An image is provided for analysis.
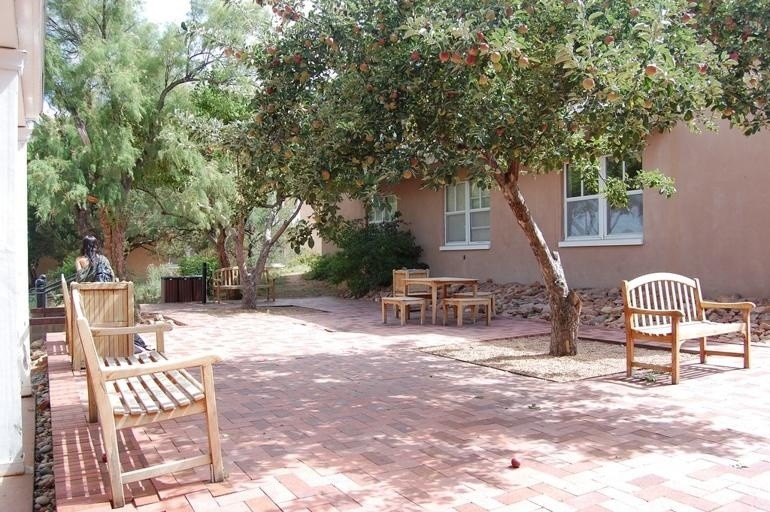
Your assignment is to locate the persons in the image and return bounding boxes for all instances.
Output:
[75,234,152,352]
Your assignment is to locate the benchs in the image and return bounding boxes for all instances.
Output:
[213,265,276,304]
[71,289,225,503]
[622,271,756,384]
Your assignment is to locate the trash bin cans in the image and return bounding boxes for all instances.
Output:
[161,275,204,302]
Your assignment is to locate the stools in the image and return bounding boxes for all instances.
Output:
[381,291,496,328]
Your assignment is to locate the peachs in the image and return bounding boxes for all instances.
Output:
[511,458,520,468]
[223,0,770,188]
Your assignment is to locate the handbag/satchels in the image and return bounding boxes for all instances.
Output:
[92,255,113,282]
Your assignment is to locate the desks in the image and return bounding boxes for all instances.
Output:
[400,277,480,325]
